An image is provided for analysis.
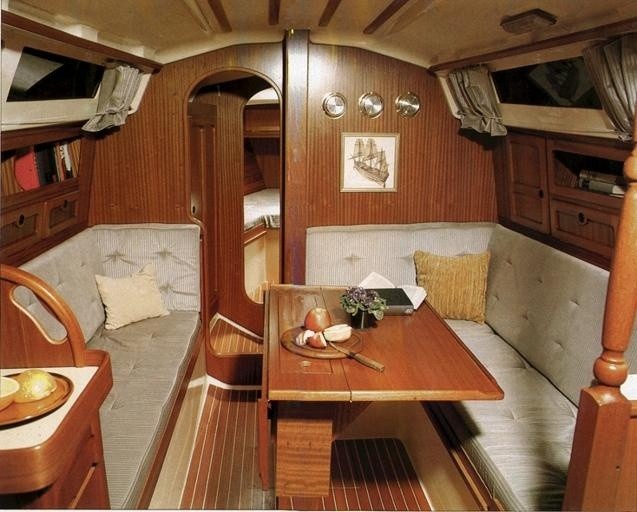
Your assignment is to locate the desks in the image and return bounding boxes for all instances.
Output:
[251,283,504,497]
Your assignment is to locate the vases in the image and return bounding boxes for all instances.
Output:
[347,309,376,329]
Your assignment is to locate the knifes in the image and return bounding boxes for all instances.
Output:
[327,341,385,373]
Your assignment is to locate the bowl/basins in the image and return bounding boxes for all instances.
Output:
[0,376,20,412]
[13,368,58,404]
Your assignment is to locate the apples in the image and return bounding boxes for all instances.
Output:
[304,308,331,333]
[295,323,352,348]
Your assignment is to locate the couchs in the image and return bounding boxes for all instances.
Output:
[0,221,206,509]
[244,187,278,247]
[305,221,636,512]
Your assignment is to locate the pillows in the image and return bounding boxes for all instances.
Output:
[411,249,489,325]
[93,264,171,329]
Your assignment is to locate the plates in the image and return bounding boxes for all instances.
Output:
[0,370,76,428]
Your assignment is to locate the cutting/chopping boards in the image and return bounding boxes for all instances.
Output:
[279,324,365,359]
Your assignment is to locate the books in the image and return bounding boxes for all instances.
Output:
[360,287,413,316]
[579,169,626,196]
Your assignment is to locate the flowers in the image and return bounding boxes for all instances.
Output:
[338,285,389,321]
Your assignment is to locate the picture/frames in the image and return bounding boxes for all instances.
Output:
[340,132,399,193]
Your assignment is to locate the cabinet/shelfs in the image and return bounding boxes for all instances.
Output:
[502,128,550,236]
[546,137,636,261]
[1,118,96,267]
[0,350,115,510]
